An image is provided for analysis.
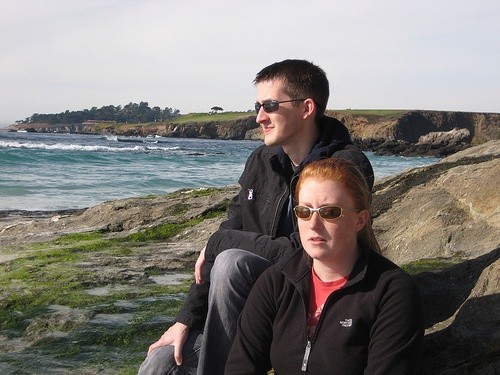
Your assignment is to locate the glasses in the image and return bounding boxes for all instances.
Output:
[293,205,361,220]
[255,98,320,114]
